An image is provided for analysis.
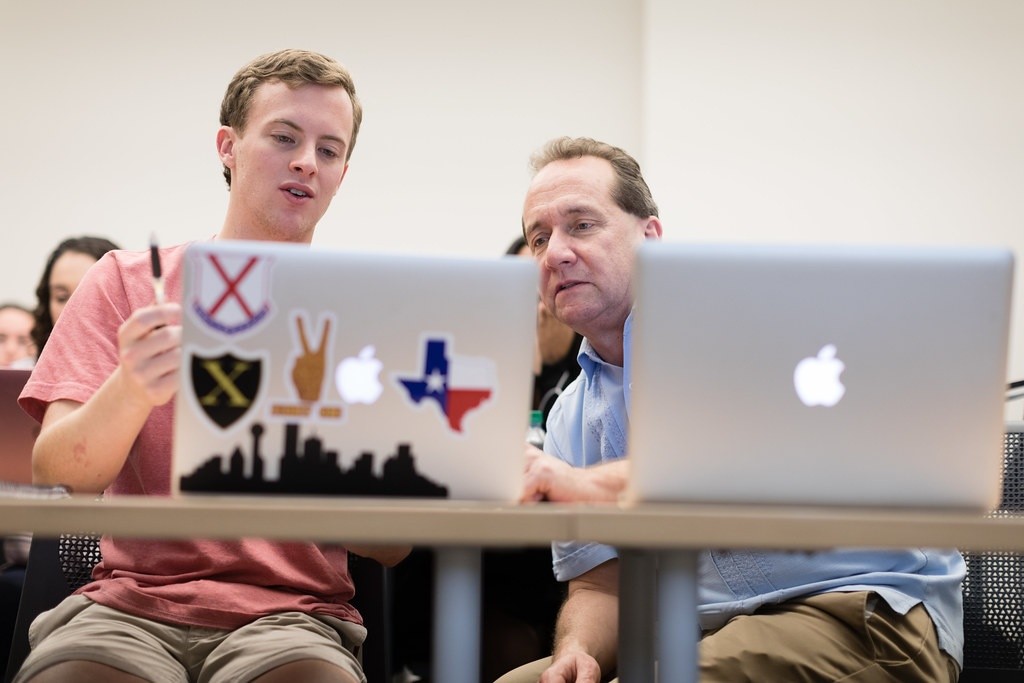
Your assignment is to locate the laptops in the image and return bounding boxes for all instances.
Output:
[0,369,42,487]
[173,245,539,504]
[632,240,1016,511]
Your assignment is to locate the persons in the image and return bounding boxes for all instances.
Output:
[502,237,585,452]
[18,49,417,683]
[30,236,122,369]
[0,305,36,370]
[490,137,969,683]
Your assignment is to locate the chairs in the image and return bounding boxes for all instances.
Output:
[5,532,104,683]
[956,425,1024,683]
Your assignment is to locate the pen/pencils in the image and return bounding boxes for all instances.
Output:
[148,232,167,332]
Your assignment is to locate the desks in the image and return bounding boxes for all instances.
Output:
[0,491,1024,683]
